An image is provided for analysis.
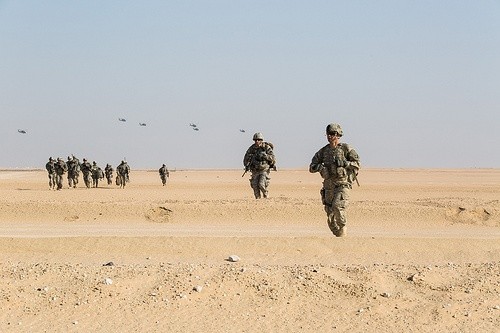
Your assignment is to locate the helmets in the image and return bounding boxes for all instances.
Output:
[327,123,343,136]
[68,156,72,159]
[57,157,61,160]
[49,157,54,160]
[253,133,263,140]
[163,164,166,166]
[122,161,124,164]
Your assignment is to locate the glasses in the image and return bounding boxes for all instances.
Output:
[327,132,339,136]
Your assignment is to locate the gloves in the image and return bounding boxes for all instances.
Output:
[336,159,347,167]
[312,162,324,172]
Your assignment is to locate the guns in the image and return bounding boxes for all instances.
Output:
[242,146,265,177]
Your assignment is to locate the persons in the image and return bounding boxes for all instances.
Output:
[243,132,276,198]
[46,157,56,191]
[55,157,67,190]
[159,163,169,186]
[309,124,359,237]
[66,155,131,189]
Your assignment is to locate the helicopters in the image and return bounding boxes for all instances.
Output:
[189,123,197,127]
[18,128,26,134]
[139,122,146,127]
[240,129,246,132]
[193,127,199,131]
[119,117,126,121]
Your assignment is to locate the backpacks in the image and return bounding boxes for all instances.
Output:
[341,143,360,183]
[263,142,275,167]
